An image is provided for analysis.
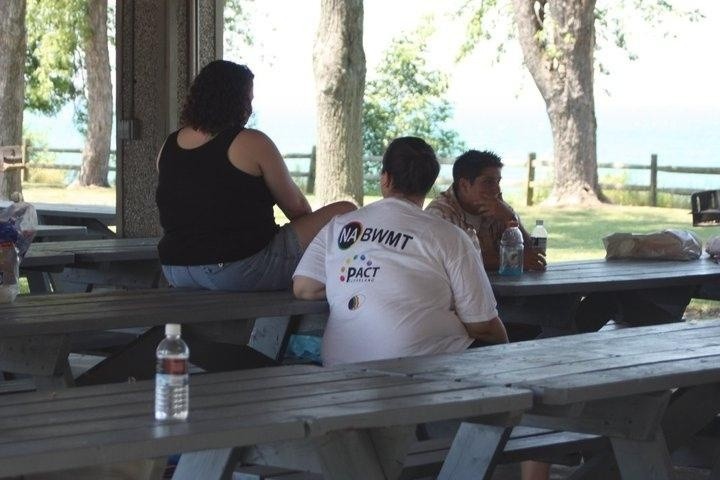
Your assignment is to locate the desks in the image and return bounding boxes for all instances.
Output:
[0,286,336,396]
[331,317,719,480]
[0,364,535,480]
[0,201,116,240]
[486,258,720,338]
[22,238,169,293]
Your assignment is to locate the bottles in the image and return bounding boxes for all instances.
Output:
[466,225,483,263]
[499,218,524,276]
[156,323,190,422]
[529,219,548,272]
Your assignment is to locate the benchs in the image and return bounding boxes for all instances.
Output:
[34,223,87,241]
[72,327,163,363]
[233,425,602,480]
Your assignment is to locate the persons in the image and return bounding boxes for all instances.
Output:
[421,148,548,274]
[156,60,358,293]
[291,136,551,479]
